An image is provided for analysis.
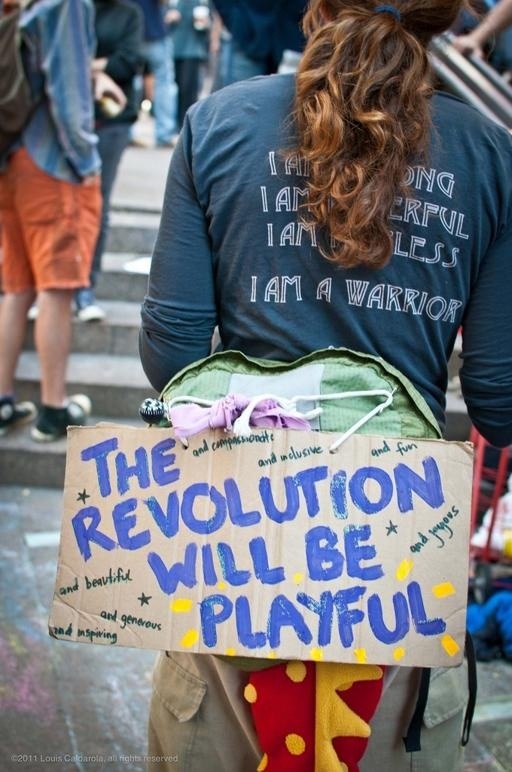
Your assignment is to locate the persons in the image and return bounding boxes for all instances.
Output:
[134,0,512,769]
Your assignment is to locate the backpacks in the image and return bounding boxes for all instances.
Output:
[0,0,41,154]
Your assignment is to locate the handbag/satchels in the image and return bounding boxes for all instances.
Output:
[154,346,443,439]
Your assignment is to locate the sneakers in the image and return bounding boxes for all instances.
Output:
[0,393,91,440]
[78,304,104,324]
